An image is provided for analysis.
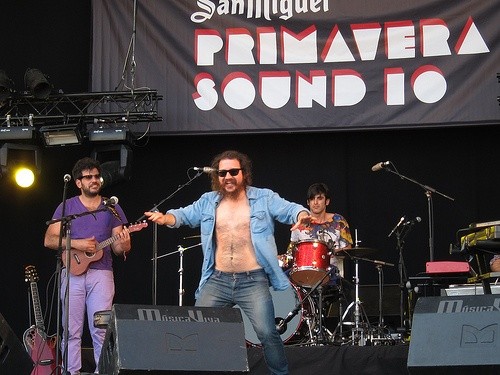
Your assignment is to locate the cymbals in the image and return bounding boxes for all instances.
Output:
[334,247,372,256]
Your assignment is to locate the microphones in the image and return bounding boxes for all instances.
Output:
[275,320,286,333]
[371,161,390,172]
[193,166,216,173]
[403,217,422,225]
[63,173,71,183]
[102,196,119,206]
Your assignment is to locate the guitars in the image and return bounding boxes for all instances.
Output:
[24,265,62,375]
[62,219,148,275]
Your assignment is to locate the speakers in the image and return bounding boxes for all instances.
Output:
[407,293,500,375]
[95,303,251,375]
[0,314,35,375]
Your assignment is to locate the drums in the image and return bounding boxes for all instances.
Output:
[290,239,330,286]
[232,280,317,348]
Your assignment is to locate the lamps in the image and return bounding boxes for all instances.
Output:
[0,69,17,110]
[0,124,136,189]
[23,65,53,97]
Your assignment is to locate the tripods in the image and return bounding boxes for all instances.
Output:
[285,253,372,346]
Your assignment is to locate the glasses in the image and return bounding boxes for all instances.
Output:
[218,169,243,177]
[79,174,100,179]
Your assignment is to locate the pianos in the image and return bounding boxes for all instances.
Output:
[426,261,477,278]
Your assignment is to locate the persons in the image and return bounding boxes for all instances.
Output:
[490,254,500,273]
[283,181,353,325]
[143,149,318,374]
[43,156,132,375]
